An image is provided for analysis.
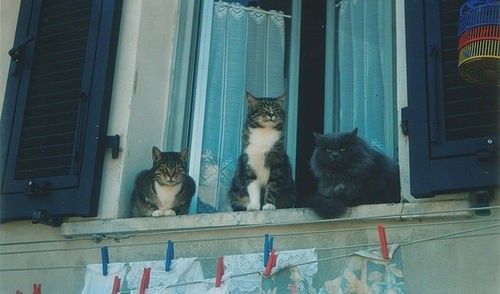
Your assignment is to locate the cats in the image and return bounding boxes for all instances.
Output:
[304,127,401,219]
[130,146,197,218]
[228,90,298,212]
